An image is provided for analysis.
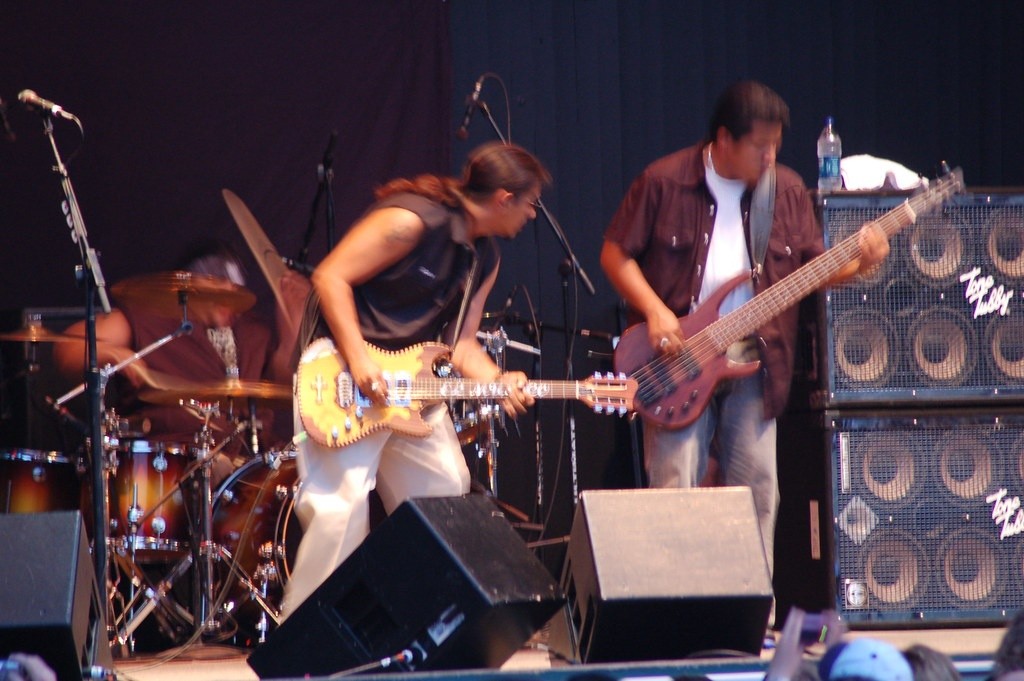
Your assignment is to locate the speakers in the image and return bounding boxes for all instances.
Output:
[246,493,566,681]
[548,486,775,671]
[0,509,117,681]
[806,185,1024,630]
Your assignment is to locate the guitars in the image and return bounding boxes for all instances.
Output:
[296,336,639,450]
[613,161,968,433]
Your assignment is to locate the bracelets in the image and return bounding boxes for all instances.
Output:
[492,368,509,382]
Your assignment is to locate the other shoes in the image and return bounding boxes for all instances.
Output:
[761,626,776,649]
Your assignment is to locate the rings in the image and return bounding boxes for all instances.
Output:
[659,338,668,347]
[371,380,380,392]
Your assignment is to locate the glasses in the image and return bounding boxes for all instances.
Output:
[507,191,541,212]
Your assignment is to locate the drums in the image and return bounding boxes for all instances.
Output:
[90,438,205,563]
[210,448,308,636]
[1,447,89,514]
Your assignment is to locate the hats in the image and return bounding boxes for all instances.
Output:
[818,638,914,681]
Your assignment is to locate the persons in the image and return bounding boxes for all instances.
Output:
[601,77,891,628]
[760,597,1024,681]
[55,253,252,649]
[277,140,552,628]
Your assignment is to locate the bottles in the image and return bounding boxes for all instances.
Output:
[817,119,843,191]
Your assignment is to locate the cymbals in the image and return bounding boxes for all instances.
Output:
[135,380,293,404]
[108,272,255,326]
[0,327,103,348]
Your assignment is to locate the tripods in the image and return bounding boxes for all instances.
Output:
[108,400,284,660]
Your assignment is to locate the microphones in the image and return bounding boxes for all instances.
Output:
[17,89,75,121]
[493,286,519,331]
[46,397,93,435]
[455,74,485,140]
[248,398,259,454]
[280,257,315,276]
